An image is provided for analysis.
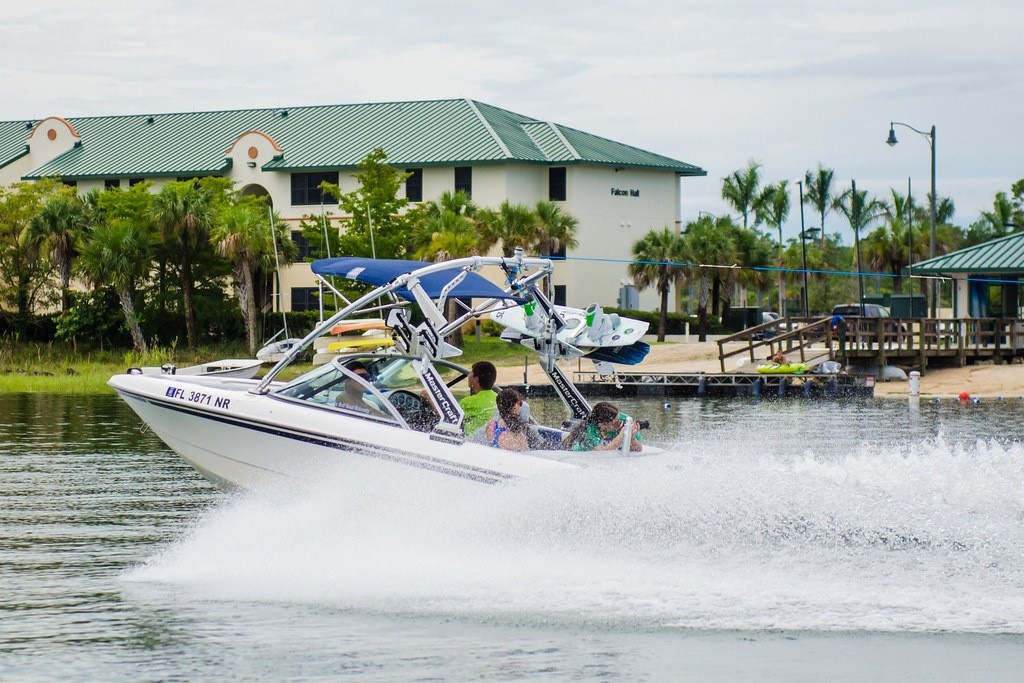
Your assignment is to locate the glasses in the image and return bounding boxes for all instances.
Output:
[358,373,371,381]
[513,401,522,406]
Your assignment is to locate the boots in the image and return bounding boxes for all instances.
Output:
[522,293,543,329]
[585,303,620,340]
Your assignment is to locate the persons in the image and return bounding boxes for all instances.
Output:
[486,388,528,450]
[561,401,643,453]
[422,361,498,436]
[333,361,396,420]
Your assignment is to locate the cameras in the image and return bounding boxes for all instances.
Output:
[636,419,651,429]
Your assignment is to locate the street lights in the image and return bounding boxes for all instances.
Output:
[875,121,936,340]
[795,178,809,319]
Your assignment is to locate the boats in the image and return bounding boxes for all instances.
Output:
[105,201,678,504]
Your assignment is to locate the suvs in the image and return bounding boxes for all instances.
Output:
[833,304,907,343]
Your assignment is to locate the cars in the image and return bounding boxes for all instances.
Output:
[762,312,797,333]
